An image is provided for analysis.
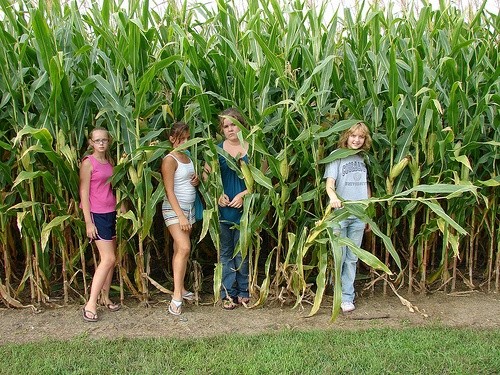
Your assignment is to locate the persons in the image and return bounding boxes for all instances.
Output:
[323,122,373,313]
[161,123,203,317]
[211,108,257,309]
[79,127,128,321]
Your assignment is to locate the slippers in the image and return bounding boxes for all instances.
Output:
[82,307,98,322]
[182,292,202,301]
[101,301,121,312]
[168,298,182,316]
[237,297,250,304]
[223,301,236,310]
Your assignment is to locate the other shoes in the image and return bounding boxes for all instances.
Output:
[340,302,356,313]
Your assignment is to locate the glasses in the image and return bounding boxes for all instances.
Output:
[91,139,110,144]
